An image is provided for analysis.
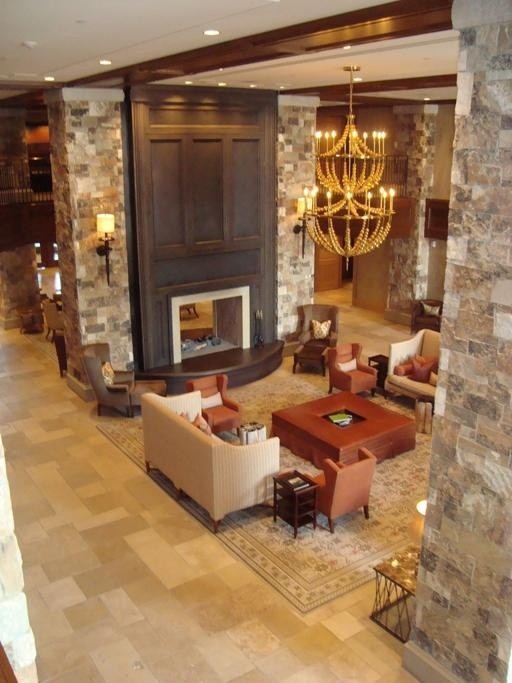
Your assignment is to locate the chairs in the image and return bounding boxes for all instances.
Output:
[412,298,443,333]
[81,346,167,417]
[293,303,339,376]
[306,446,377,533]
[42,298,64,343]
[187,374,242,439]
[327,341,378,395]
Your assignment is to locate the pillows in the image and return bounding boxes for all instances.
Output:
[338,358,358,372]
[423,304,441,317]
[201,392,223,410]
[395,353,437,386]
[102,363,114,386]
[192,414,211,435]
[310,320,331,339]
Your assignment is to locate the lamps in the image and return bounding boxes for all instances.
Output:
[295,196,313,257]
[96,213,115,285]
[296,67,398,273]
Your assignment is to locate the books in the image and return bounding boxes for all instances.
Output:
[339,414,353,426]
[329,413,349,422]
[283,474,310,492]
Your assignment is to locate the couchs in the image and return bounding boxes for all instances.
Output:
[384,328,441,404]
[140,391,281,533]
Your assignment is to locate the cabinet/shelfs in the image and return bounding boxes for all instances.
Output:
[272,469,320,539]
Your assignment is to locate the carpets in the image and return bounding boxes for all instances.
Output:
[94,370,437,614]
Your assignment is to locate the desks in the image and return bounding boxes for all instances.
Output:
[371,546,423,642]
